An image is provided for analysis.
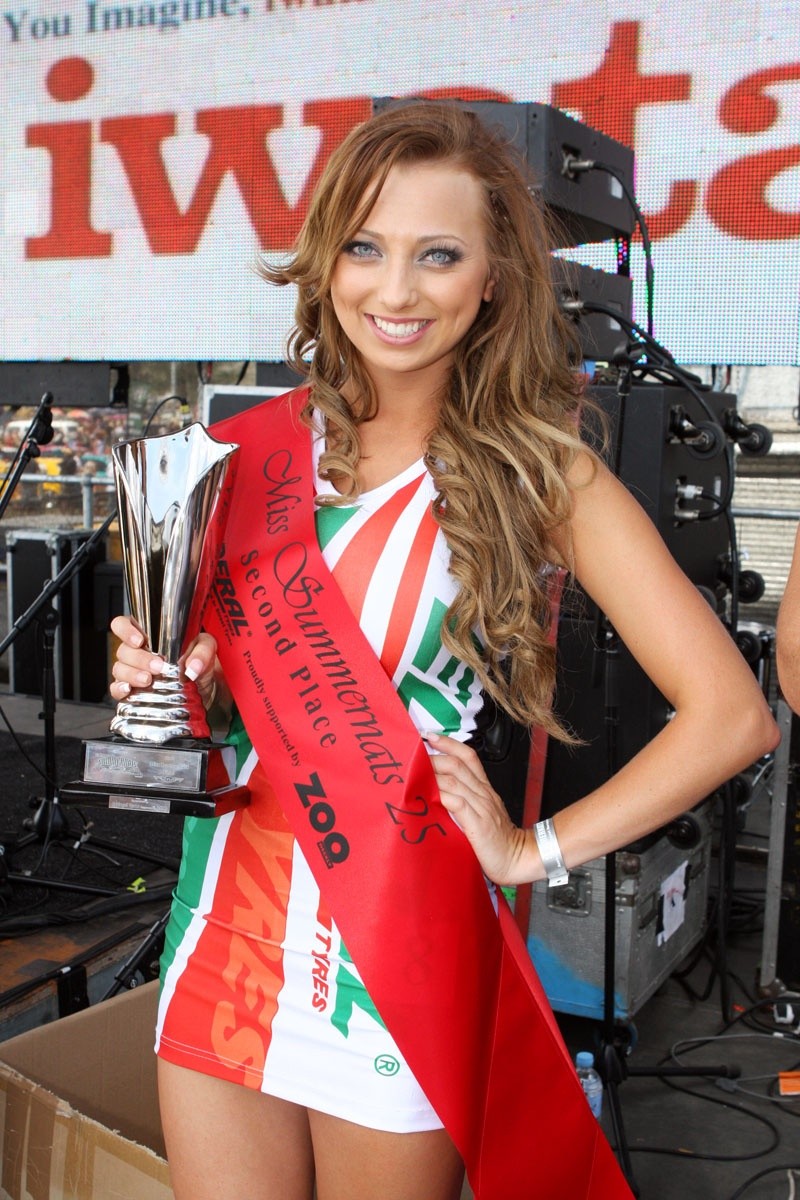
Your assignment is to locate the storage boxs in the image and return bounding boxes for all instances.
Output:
[0,972,180,1200]
[4,523,106,703]
[500,787,713,1026]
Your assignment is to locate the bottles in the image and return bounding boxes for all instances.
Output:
[575,1051,602,1124]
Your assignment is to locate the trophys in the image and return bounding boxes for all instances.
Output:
[59,420,257,818]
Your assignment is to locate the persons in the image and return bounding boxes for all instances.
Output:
[775,525,800,714]
[105,107,781,1200]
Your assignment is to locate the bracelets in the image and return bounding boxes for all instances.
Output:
[533,817,569,890]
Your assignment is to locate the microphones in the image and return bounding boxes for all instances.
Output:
[32,392,54,445]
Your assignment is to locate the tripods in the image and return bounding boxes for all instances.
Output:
[593,851,743,1200]
[0,512,182,880]
